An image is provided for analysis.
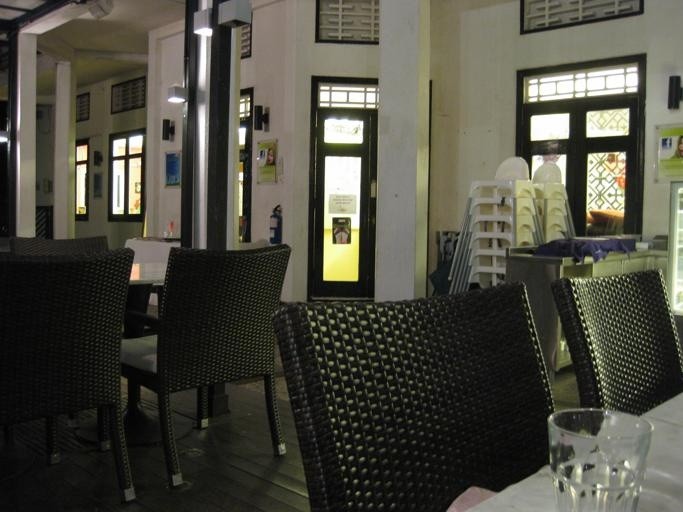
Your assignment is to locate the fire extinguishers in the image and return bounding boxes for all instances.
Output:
[270,204,282,244]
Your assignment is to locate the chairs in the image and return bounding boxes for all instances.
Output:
[0,248,135,502]
[270,279,556,511]
[7,233,109,254]
[547,267,683,417]
[121,243,292,488]
[446,156,577,296]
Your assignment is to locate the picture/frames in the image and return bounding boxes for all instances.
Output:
[75,90,91,124]
[314,2,379,44]
[236,22,252,61]
[517,1,645,36]
[109,73,149,115]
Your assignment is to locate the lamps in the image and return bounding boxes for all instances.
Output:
[91,149,103,168]
[160,118,177,142]
[667,75,682,111]
[223,3,256,36]
[167,83,187,104]
[254,103,270,131]
[192,8,213,42]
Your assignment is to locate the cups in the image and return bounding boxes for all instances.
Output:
[548,408,652,512]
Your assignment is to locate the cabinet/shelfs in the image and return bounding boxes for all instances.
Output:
[507,227,671,372]
